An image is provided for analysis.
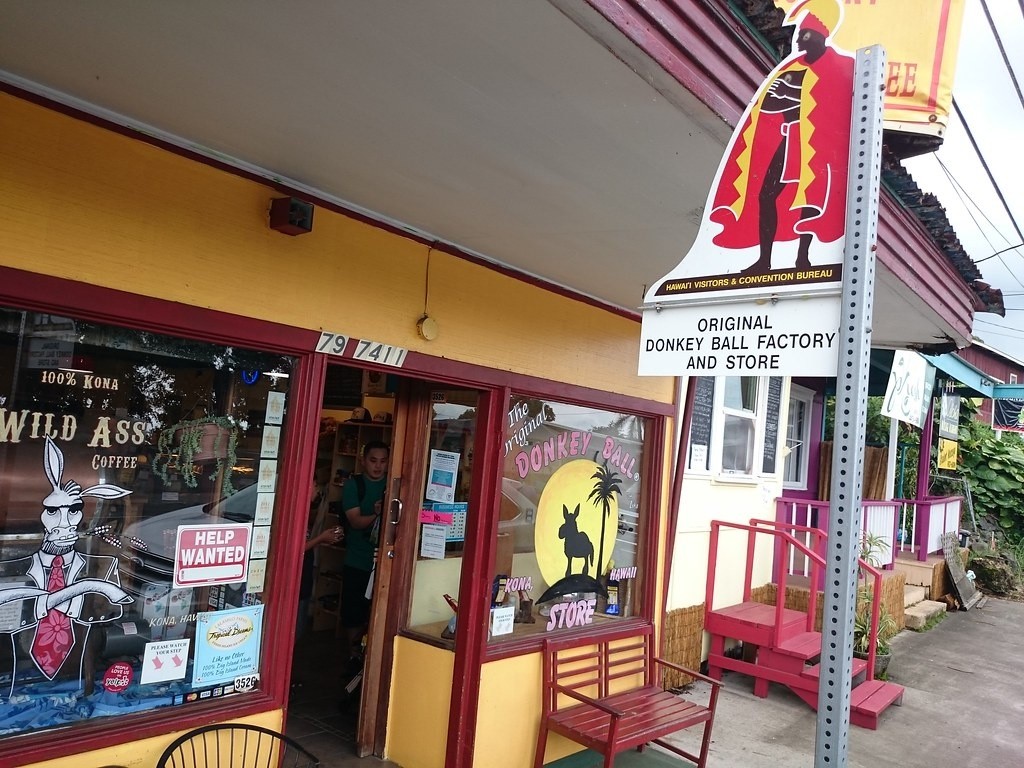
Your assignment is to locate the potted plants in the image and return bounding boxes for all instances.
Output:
[152,415,243,497]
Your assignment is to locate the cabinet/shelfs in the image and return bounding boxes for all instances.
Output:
[311,420,471,639]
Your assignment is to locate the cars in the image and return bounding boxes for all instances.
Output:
[116,482,257,607]
[497,478,542,553]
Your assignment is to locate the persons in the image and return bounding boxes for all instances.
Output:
[132,440,170,522]
[290,471,346,680]
[338,439,390,686]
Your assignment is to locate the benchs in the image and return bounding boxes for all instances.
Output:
[533,621,727,768]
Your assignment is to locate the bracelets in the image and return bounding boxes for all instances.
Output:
[373,512,378,516]
